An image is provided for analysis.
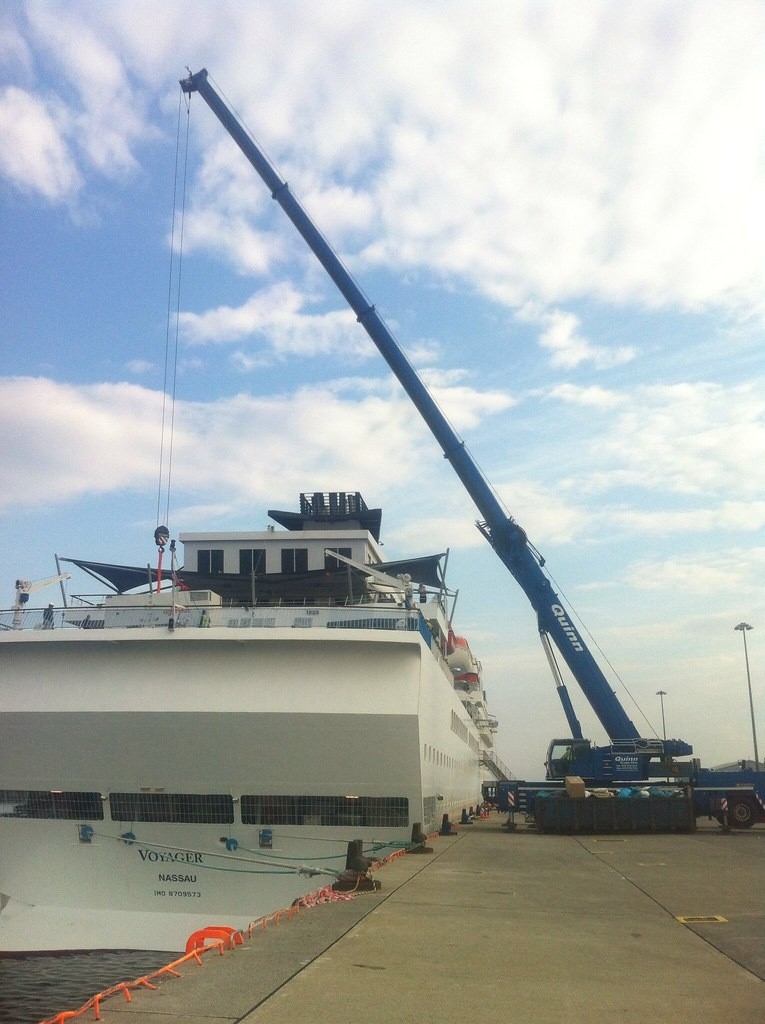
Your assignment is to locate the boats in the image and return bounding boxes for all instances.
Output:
[454,656,482,685]
[1,493,500,959]
[446,636,472,677]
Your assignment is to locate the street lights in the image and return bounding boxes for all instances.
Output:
[734,622,759,773]
[656,690,668,741]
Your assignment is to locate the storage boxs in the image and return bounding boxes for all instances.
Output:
[565,775,585,798]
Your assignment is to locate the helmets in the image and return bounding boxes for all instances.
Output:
[49,602,54,605]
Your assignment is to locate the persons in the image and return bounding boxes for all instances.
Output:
[559,747,572,761]
[417,583,427,603]
[42,604,54,629]
[199,610,212,628]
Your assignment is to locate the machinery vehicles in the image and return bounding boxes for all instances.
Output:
[153,65,765,837]
